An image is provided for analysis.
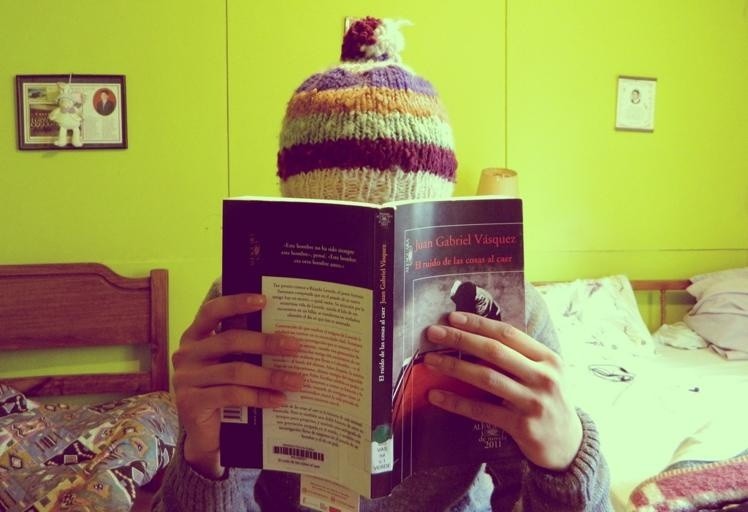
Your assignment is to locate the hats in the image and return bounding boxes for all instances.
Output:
[277,17,457,204]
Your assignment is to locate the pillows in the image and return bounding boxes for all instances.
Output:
[683,266,748,362]
[537,273,657,368]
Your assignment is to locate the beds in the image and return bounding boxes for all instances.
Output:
[0,260,185,512]
[529,278,748,511]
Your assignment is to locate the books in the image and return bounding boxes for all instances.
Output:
[217,193,529,502]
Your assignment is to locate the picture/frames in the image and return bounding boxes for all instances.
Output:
[614,75,658,133]
[14,73,128,151]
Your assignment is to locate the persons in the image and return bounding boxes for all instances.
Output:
[30,112,59,135]
[148,15,618,512]
[97,90,113,114]
